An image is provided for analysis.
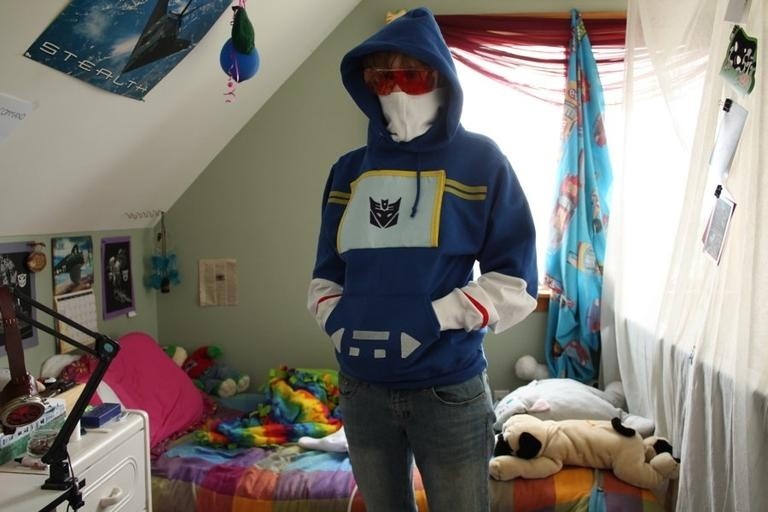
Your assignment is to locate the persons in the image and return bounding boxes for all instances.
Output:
[305,7,538,512]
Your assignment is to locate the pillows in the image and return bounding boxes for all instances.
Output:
[62,331,221,462]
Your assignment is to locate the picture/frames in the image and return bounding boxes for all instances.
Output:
[101,238,136,321]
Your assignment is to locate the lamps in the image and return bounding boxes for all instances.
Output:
[0,285,121,491]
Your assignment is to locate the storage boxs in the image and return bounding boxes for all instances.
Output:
[53,381,87,421]
[0,395,67,468]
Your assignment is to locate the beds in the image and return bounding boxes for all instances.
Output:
[152,376,672,511]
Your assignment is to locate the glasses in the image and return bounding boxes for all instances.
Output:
[363,65,444,96]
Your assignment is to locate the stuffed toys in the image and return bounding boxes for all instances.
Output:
[488,413,681,489]
[493,377,655,440]
[161,344,250,399]
[515,355,549,381]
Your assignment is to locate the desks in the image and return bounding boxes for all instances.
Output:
[0,409,152,512]
